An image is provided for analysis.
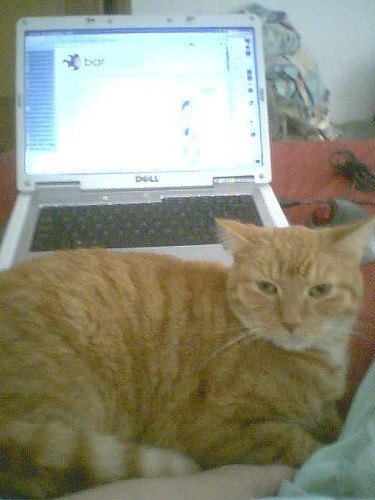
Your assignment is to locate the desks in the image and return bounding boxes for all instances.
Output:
[1,140,374,417]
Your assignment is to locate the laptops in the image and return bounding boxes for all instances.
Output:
[0,15,289,274]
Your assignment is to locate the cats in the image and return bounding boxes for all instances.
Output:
[0,217,374,499]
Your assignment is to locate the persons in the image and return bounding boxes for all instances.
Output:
[59,464,300,500]
